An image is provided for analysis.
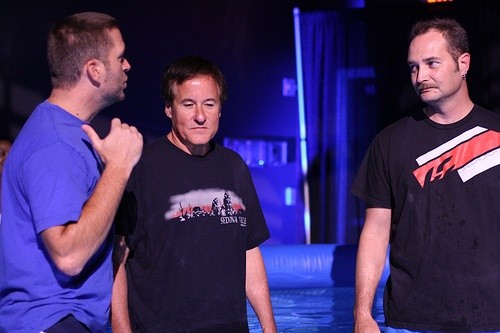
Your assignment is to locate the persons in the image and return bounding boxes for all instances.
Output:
[0,131,17,179]
[349,18,500,333]
[1,11,145,333]
[108,54,278,333]
